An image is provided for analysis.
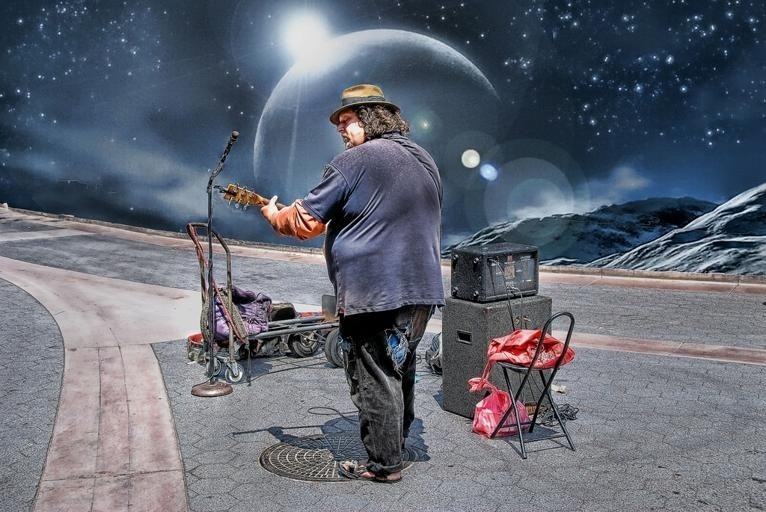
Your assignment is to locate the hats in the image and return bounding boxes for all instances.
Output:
[328,84,401,125]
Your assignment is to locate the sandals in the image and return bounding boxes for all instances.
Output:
[339,460,403,483]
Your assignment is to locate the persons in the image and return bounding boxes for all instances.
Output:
[257,78,447,484]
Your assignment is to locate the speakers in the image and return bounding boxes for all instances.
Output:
[451,241,539,303]
[442,296,552,422]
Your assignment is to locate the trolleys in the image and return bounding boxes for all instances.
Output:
[184,219,350,384]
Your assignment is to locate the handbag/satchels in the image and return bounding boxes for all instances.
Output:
[475,327,575,394]
